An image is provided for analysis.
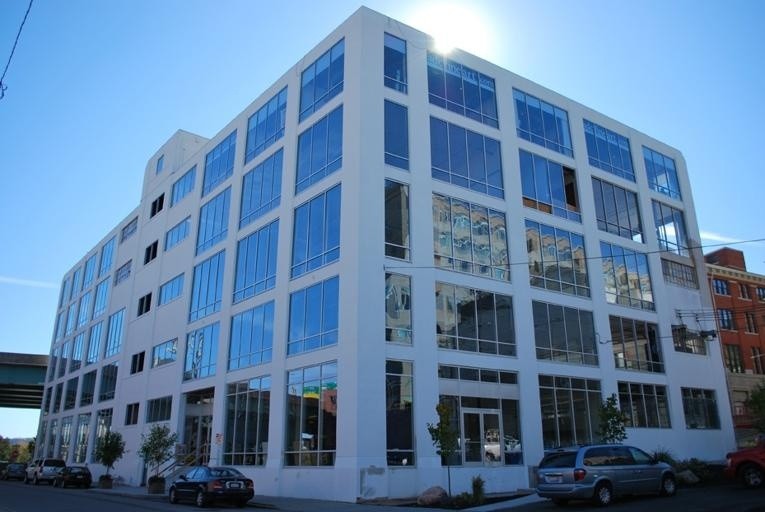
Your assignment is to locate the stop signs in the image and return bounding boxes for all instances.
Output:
[12,451,18,457]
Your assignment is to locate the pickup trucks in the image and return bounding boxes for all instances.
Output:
[458,429,521,461]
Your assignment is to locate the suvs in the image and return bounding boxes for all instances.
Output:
[25,457,66,485]
[535,443,678,505]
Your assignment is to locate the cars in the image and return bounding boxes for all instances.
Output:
[245,447,262,456]
[0,463,24,480]
[0,460,9,469]
[168,466,253,507]
[52,465,91,489]
[722,443,765,488]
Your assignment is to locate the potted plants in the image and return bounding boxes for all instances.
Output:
[136,422,182,494]
[92,428,129,488]
